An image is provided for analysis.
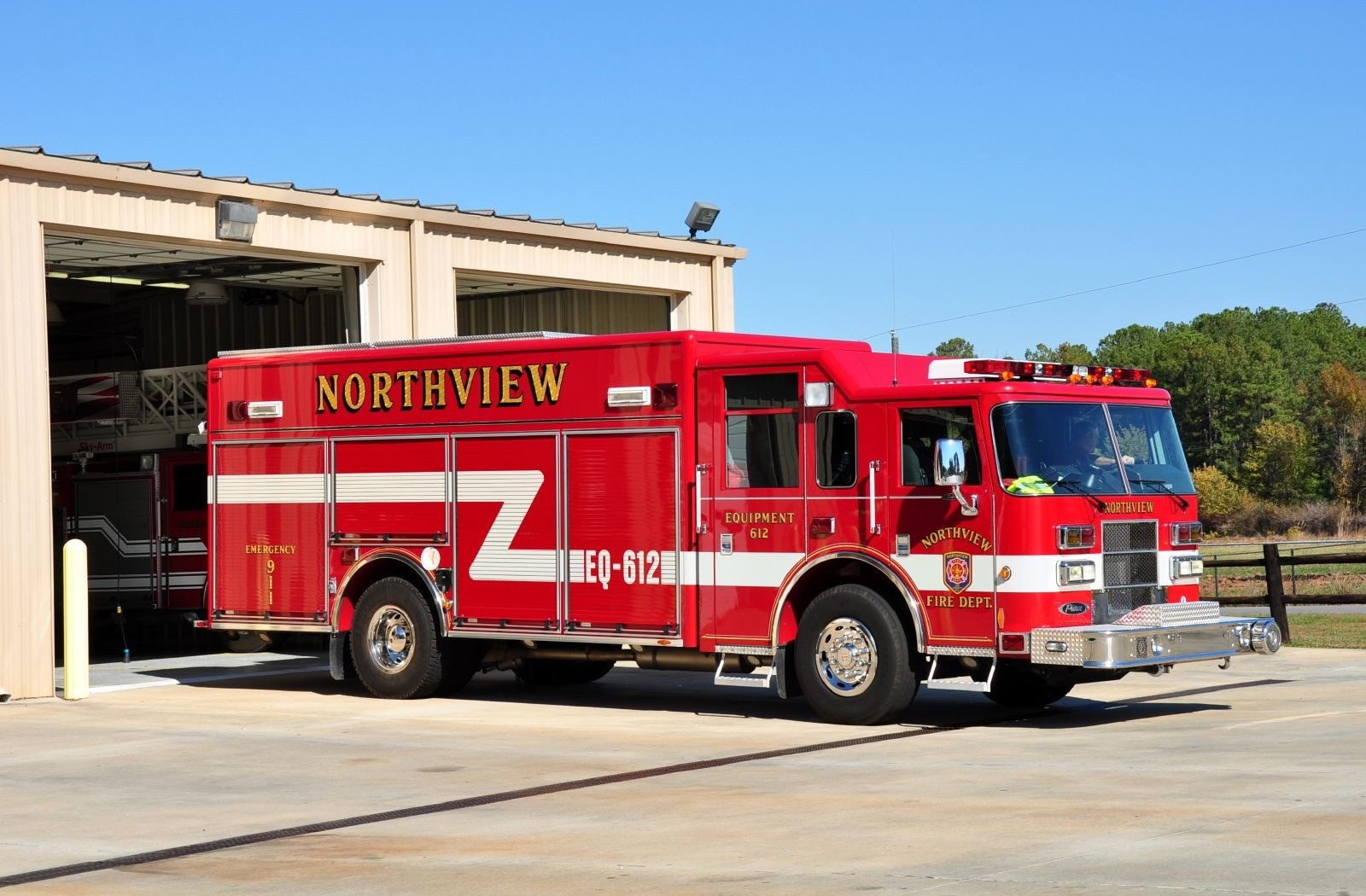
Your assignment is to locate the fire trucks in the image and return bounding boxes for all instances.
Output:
[193,330,1281,727]
[51,364,278,654]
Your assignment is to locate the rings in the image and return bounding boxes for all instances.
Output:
[1126,459,1129,461]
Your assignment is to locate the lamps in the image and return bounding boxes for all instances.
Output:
[216,199,258,243]
[684,201,720,242]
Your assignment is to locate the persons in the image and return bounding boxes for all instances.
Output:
[1059,421,1135,466]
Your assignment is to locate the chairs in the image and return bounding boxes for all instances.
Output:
[969,453,1006,484]
[903,444,925,486]
